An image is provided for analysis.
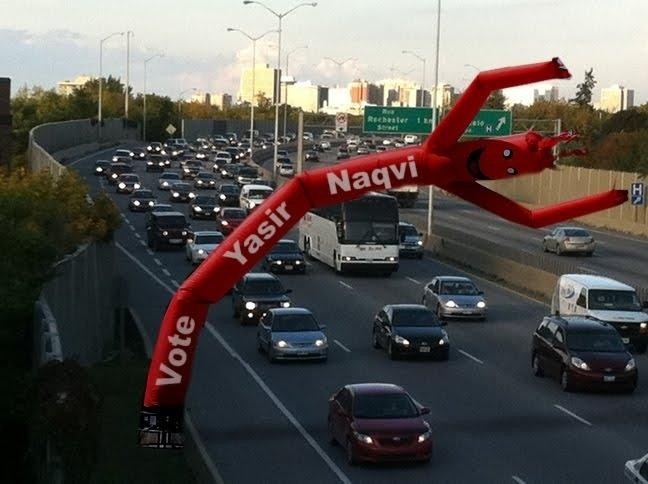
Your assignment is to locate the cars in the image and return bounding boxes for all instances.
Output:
[232,273,293,326]
[327,383,434,465]
[543,226,597,255]
[421,276,488,323]
[371,301,450,362]
[255,305,329,365]
[623,453,648,483]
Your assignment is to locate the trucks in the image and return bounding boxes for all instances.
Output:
[550,274,647,346]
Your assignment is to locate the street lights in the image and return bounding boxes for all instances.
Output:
[228,27,283,160]
[99,31,124,143]
[123,28,138,119]
[388,67,408,106]
[267,44,309,150]
[143,53,165,141]
[243,1,319,186]
[402,49,426,108]
[463,63,481,74]
[178,87,198,134]
[324,55,357,113]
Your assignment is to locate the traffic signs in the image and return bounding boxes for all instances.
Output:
[362,104,439,133]
[442,106,512,137]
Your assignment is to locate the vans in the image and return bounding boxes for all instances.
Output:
[530,313,638,394]
